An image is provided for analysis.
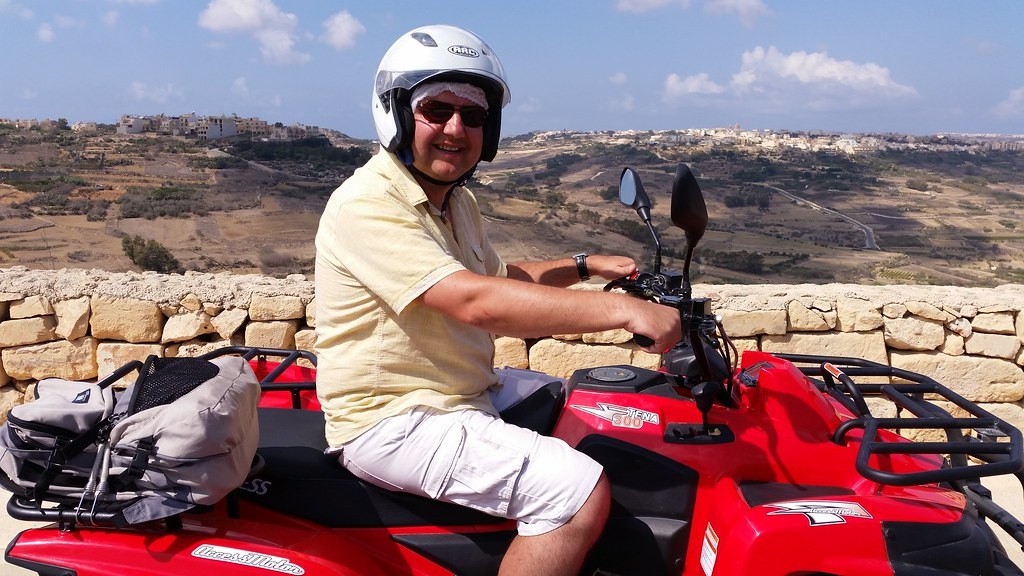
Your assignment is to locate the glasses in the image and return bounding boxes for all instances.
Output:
[418,100,488,129]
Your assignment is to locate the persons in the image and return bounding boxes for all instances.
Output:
[316,24,681,576]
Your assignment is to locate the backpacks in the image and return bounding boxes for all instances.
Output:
[0,354,261,526]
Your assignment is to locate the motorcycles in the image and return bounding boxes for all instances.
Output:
[0,163,1024,576]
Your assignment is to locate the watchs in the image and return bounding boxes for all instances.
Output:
[572,252,591,282]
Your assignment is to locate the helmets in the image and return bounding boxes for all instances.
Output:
[372,24,505,165]
